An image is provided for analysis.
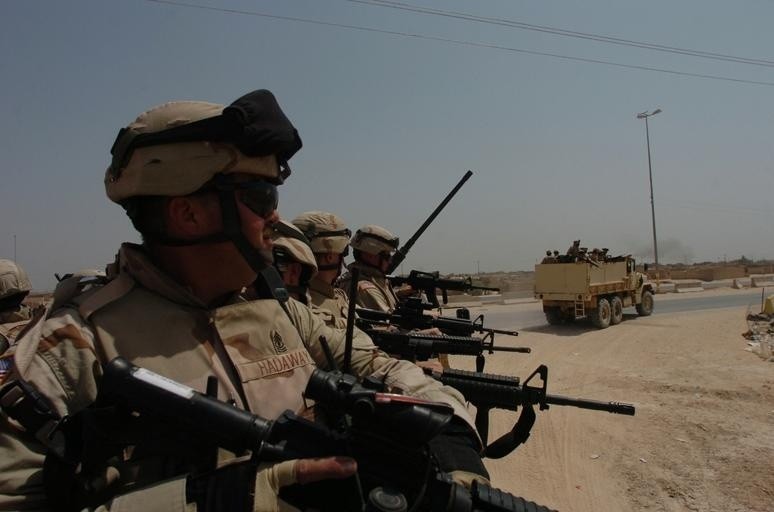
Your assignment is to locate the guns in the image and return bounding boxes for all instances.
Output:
[352,297,521,340]
[348,318,532,359]
[46,356,559,511]
[384,271,502,311]
[415,362,634,415]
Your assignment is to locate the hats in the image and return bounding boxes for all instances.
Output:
[290,210,352,255]
[349,223,400,256]
[104,88,304,205]
[0,256,35,300]
[272,217,319,284]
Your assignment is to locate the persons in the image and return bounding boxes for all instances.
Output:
[541,240,608,264]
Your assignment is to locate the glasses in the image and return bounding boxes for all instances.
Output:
[185,181,278,219]
[273,250,294,266]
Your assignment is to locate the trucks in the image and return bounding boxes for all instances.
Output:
[531,254,655,330]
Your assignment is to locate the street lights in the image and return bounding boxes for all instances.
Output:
[637,110,663,281]
